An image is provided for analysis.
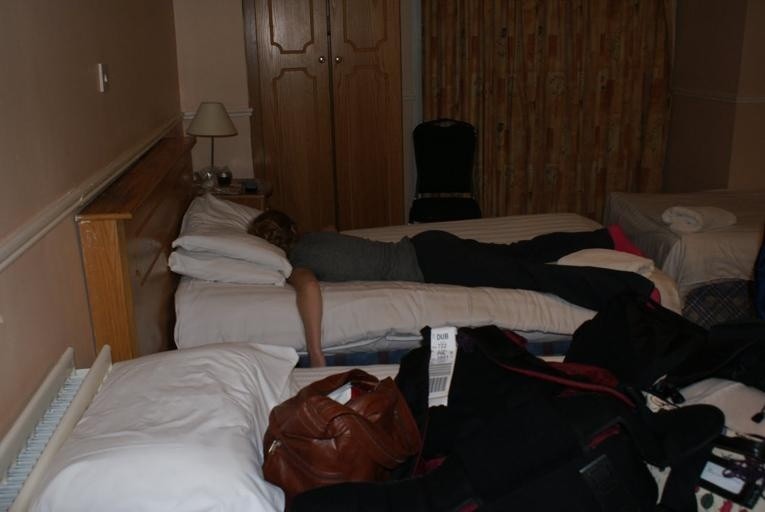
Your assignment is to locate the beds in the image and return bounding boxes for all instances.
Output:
[606,187,765,290]
[75,134,681,356]
[1,342,763,507]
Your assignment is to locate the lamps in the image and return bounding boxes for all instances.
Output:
[189,98,237,184]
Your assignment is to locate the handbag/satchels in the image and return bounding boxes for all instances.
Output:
[262,369,422,496]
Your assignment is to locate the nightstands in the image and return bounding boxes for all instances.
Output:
[198,175,269,211]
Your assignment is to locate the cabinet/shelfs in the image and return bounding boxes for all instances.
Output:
[241,0,406,234]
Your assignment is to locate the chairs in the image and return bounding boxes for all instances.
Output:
[407,117,485,224]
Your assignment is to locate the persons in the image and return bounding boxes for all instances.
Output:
[246,207,662,368]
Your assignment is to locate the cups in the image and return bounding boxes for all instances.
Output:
[214,166,232,186]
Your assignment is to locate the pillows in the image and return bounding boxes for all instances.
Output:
[163,243,286,289]
[174,186,297,270]
[30,340,304,509]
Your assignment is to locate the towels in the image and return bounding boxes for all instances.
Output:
[662,206,737,235]
